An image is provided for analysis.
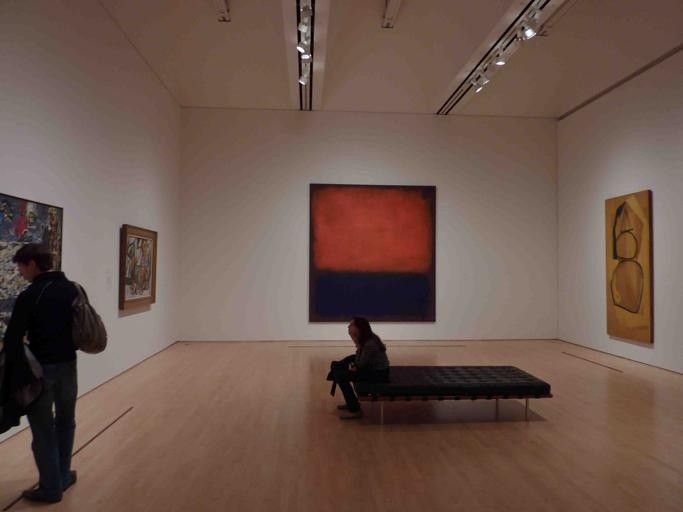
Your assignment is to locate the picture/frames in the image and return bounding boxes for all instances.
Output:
[119,223,158,310]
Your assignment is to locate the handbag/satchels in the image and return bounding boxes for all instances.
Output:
[67,280,107,354]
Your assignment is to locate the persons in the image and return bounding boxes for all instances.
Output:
[335,314,391,421]
[3,243,88,504]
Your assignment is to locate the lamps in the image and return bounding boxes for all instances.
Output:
[296,0,315,111]
[435,0,551,115]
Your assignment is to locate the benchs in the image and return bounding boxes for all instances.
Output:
[357,365,553,425]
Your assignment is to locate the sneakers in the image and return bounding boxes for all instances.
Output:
[62,469,78,492]
[22,487,64,504]
[337,404,351,409]
[340,411,364,419]
